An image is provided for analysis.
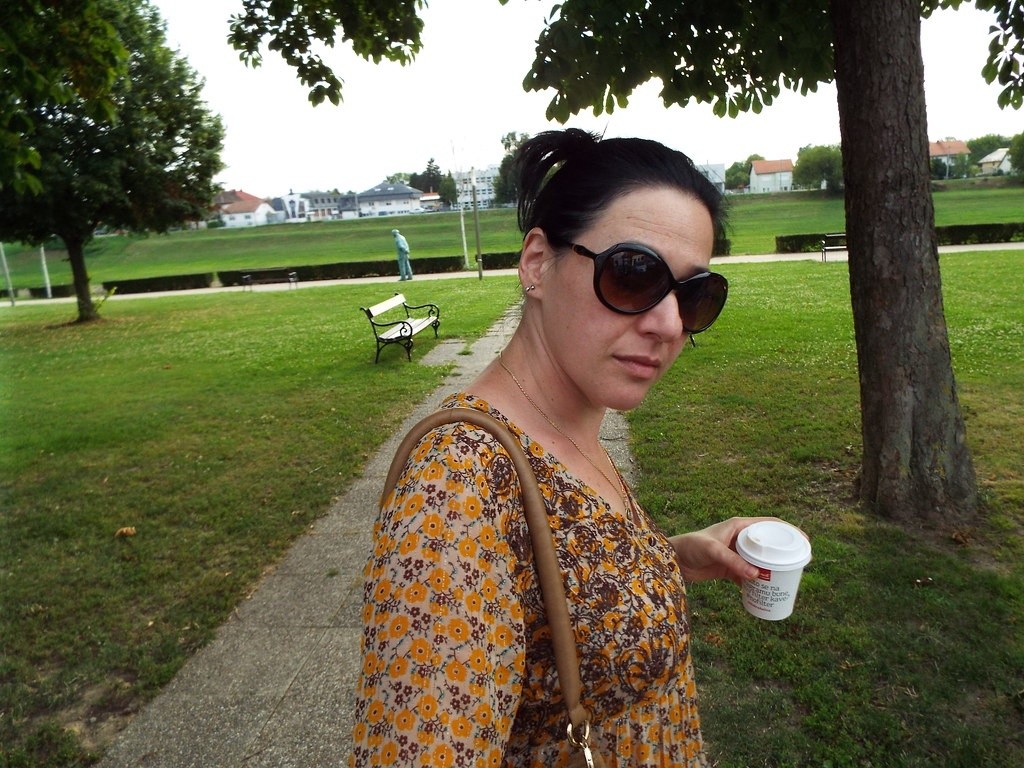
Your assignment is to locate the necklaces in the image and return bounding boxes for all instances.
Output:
[499,352,633,521]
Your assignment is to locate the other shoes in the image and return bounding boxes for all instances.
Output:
[399,279,407,281]
[407,277,413,280]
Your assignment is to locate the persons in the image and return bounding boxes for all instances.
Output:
[351,127,810,768]
[392,229,412,281]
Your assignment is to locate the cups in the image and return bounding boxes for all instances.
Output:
[735,521,812,621]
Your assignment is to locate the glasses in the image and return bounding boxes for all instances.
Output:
[548,239,729,334]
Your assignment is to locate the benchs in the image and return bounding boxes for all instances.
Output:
[360,292,440,364]
[822,234,847,263]
[239,267,300,293]
